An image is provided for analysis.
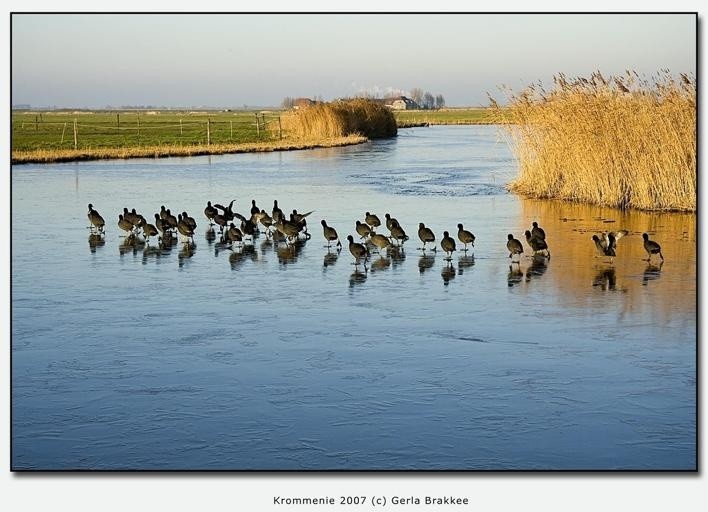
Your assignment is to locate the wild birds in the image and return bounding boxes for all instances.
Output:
[370,253,391,278]
[507,222,546,259]
[643,233,664,260]
[118,206,197,241]
[591,230,628,256]
[642,260,664,286]
[458,224,476,250]
[418,249,435,275]
[458,249,475,275]
[418,223,435,249]
[324,247,342,273]
[88,203,105,233]
[321,220,341,247]
[441,258,456,287]
[508,260,523,287]
[204,200,315,244]
[441,231,456,258]
[347,212,408,264]
[348,264,368,296]
